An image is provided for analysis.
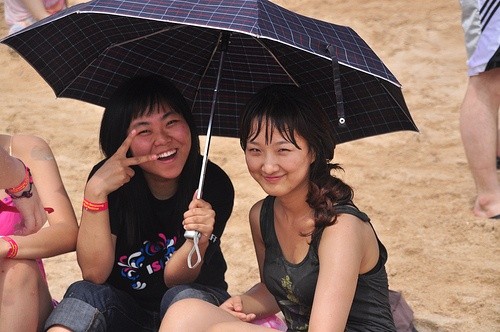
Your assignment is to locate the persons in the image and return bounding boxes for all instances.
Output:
[0,147,48,236]
[460,0,500,220]
[4,0,68,52]
[0,135,79,331]
[43,78,235,331]
[159,84,396,332]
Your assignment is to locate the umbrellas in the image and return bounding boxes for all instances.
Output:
[0,0,421,270]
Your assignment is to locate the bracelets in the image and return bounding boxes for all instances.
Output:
[8,158,28,193]
[82,199,109,212]
[2,236,18,258]
[5,166,33,199]
